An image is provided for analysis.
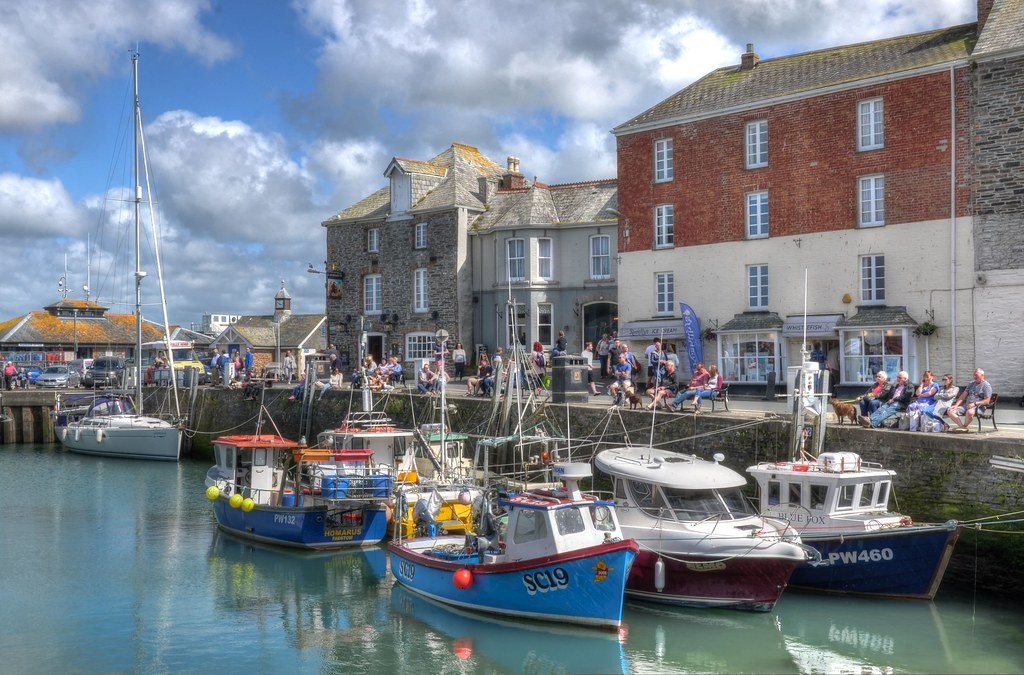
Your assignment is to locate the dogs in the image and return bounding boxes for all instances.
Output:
[624,391,642,410]
[827,398,858,425]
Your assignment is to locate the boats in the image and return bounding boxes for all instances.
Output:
[455,241,594,514]
[387,323,635,632]
[205,381,396,552]
[295,317,491,541]
[585,325,821,612]
[672,266,964,601]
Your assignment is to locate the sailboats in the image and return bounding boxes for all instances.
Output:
[50,40,183,461]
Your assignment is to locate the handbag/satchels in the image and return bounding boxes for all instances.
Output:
[881,411,903,428]
[630,355,641,372]
[899,409,942,432]
[541,372,550,388]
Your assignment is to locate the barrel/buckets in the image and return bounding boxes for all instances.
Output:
[320,475,350,498]
[367,475,393,497]
[281,494,304,507]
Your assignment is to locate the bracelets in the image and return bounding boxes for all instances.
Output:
[974,402,977,406]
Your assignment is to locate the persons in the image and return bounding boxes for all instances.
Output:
[284,350,295,384]
[2,360,19,391]
[209,346,254,387]
[145,356,170,387]
[349,331,568,400]
[288,343,344,403]
[581,321,722,414]
[18,367,29,390]
[811,342,993,434]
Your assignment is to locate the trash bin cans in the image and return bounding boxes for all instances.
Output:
[305,353,331,384]
[552,355,589,402]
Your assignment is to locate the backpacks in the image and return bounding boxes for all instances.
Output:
[533,351,546,371]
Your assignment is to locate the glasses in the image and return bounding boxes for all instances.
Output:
[897,376,902,379]
[618,357,621,358]
[942,379,948,381]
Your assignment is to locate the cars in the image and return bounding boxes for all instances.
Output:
[66,358,94,386]
[35,365,81,388]
[85,355,124,389]
[0,365,43,390]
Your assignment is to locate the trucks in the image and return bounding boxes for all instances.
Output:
[134,339,205,385]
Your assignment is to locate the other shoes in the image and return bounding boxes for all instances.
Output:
[952,427,969,433]
[645,406,651,411]
[665,402,675,412]
[537,386,541,394]
[318,397,321,400]
[691,402,698,405]
[858,415,871,428]
[594,392,601,396]
[462,393,472,396]
[695,411,703,414]
[289,395,295,400]
[944,423,950,432]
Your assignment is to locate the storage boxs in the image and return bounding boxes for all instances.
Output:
[433,549,500,565]
[368,474,394,497]
[281,494,303,507]
[322,475,349,498]
[343,513,361,527]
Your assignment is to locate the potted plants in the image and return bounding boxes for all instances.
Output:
[701,327,716,342]
[913,321,938,340]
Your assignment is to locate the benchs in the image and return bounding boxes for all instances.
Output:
[680,382,730,412]
[472,375,496,395]
[902,393,998,432]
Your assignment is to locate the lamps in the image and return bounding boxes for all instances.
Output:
[308,268,327,275]
[606,207,629,238]
